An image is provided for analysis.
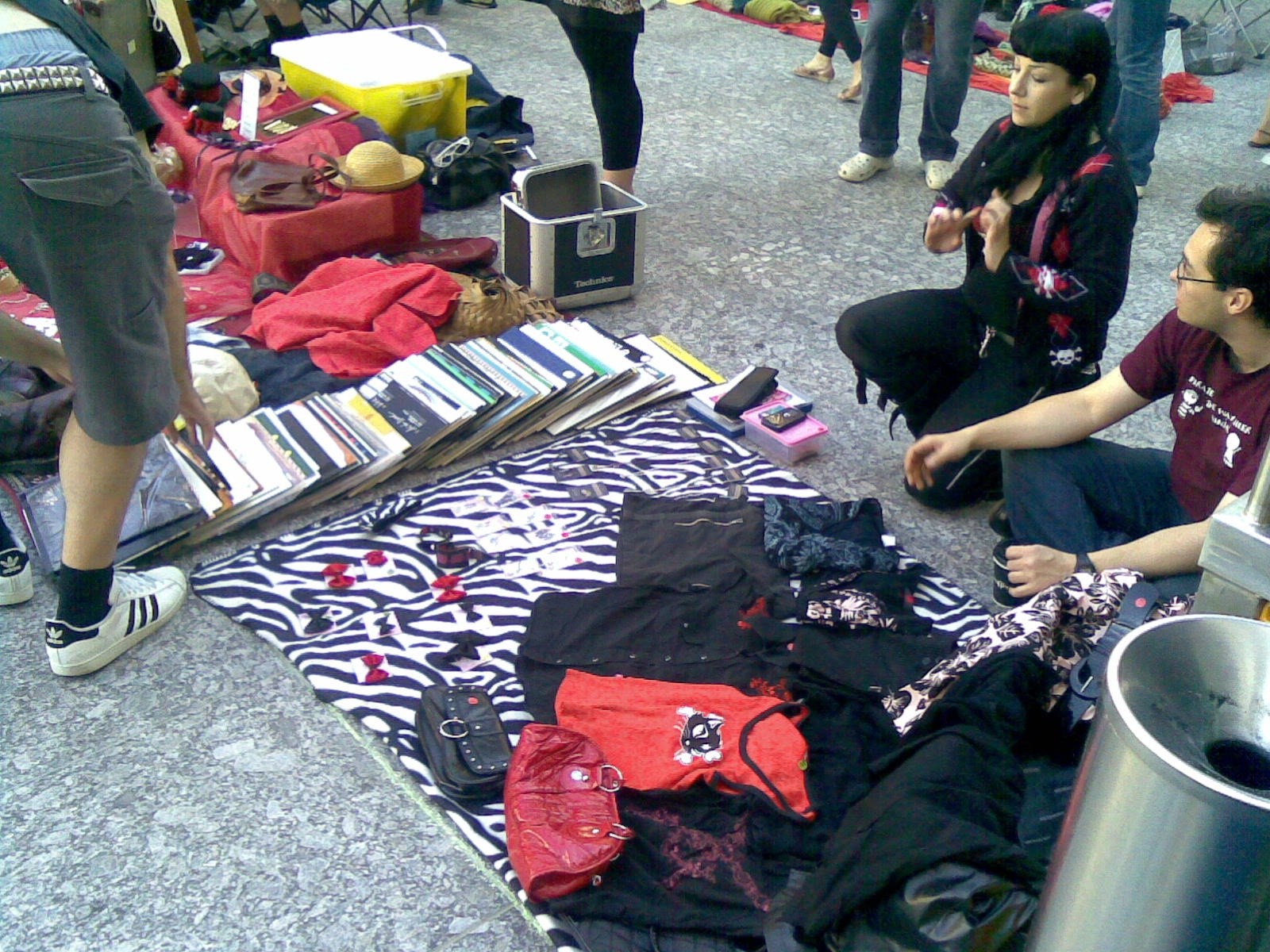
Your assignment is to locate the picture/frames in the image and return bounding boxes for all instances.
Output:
[257,95,359,146]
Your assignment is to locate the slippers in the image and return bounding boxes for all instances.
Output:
[1248,141,1270,149]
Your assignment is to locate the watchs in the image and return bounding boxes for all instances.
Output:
[1074,551,1097,573]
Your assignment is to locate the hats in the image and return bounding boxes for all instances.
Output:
[324,141,425,193]
[228,68,282,109]
[165,62,232,108]
[182,103,232,140]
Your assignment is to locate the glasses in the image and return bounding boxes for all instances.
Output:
[434,137,470,167]
[1176,258,1228,286]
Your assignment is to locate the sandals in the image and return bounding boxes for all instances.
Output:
[838,150,894,183]
[922,158,956,189]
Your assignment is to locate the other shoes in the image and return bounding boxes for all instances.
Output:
[838,78,863,100]
[988,497,1012,538]
[1135,184,1145,197]
[793,64,835,82]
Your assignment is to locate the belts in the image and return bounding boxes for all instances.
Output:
[0,65,111,95]
[1048,583,1161,749]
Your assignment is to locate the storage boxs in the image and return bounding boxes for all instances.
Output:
[500,157,647,310]
[272,28,473,154]
[740,400,828,465]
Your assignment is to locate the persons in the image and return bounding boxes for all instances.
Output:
[904,184,1269,601]
[793,0,864,101]
[834,11,1138,511]
[0,1,215,676]
[1101,0,1172,197]
[250,0,311,67]
[549,0,644,195]
[1248,92,1270,150]
[838,0,986,190]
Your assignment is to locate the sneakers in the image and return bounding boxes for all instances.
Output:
[0,527,34,605]
[235,29,284,67]
[45,566,186,677]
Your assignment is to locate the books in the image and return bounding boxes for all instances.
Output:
[18,315,813,578]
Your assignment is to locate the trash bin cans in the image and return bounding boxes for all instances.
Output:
[1028,613,1270,951]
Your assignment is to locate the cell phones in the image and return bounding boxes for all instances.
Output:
[762,407,806,432]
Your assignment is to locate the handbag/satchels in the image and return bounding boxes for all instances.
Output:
[419,135,508,210]
[228,150,350,212]
[415,684,513,800]
[504,724,635,901]
